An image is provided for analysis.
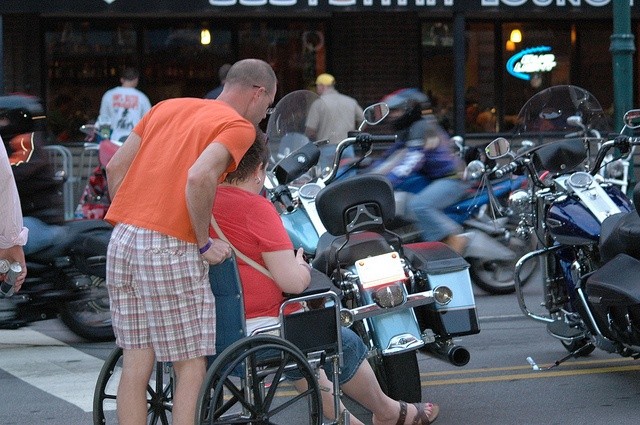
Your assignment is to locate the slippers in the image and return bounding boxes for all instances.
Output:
[397,401,438,424]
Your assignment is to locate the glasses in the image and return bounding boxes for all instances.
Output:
[252,85,277,114]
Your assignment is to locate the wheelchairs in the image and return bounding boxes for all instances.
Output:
[93,240,353,425]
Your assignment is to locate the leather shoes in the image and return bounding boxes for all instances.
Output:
[445,237,470,256]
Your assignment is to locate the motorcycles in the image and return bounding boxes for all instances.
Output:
[320,157,541,296]
[0,217,116,341]
[563,115,640,198]
[70,122,126,221]
[253,87,482,404]
[486,82,640,371]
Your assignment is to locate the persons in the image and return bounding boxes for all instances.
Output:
[303,75,365,178]
[375,88,474,259]
[85,64,152,168]
[0,134,29,294]
[203,62,234,99]
[209,132,439,425]
[104,59,279,425]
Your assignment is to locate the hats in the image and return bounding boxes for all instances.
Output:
[316,73,335,85]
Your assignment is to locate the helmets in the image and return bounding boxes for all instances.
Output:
[384,89,432,131]
